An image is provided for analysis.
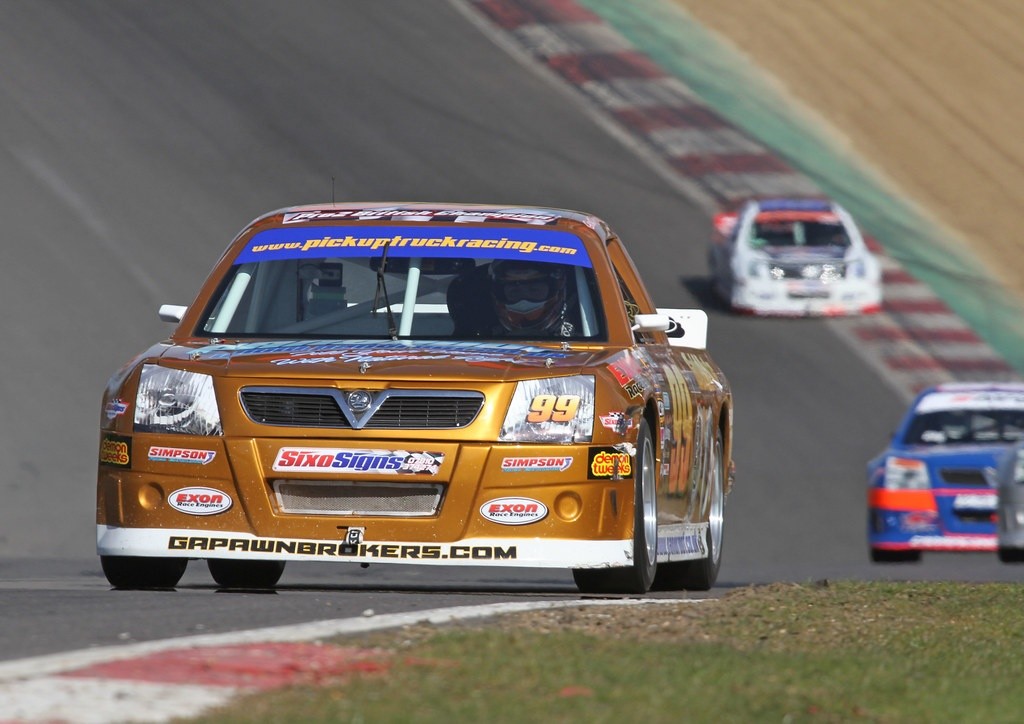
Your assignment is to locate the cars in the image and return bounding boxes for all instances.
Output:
[95,203,732,593]
[866,381,1024,564]
[707,198,884,320]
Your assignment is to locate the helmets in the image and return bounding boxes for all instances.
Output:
[487,259,576,334]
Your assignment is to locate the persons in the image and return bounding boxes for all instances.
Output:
[478,255,583,338]
